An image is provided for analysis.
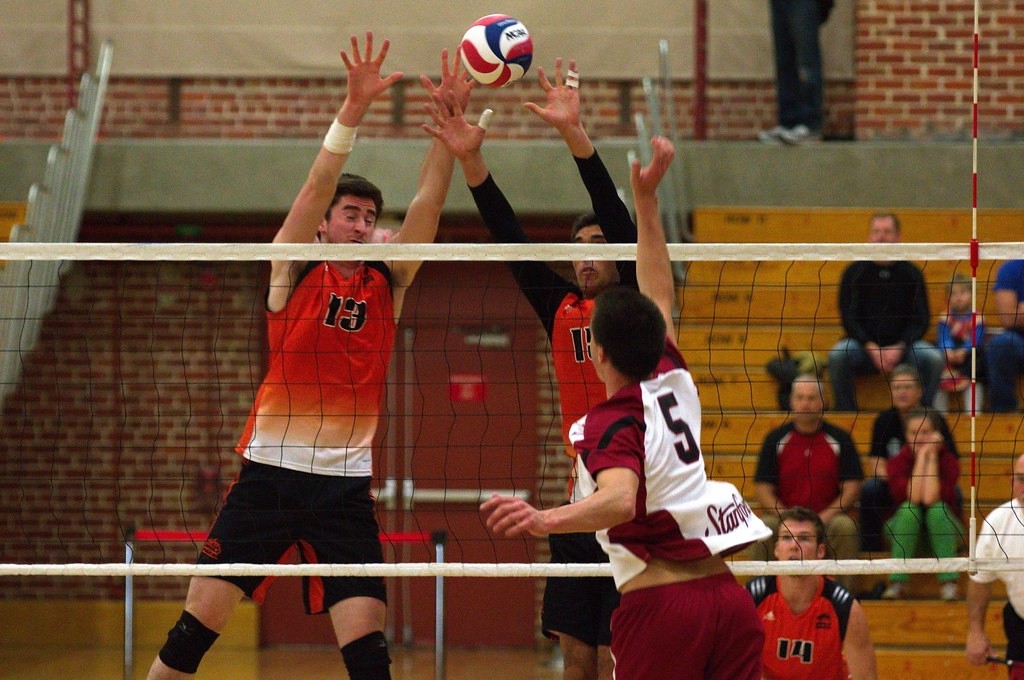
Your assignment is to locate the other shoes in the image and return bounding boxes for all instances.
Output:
[883,583,900,598]
[758,125,789,144]
[941,582,957,600]
[779,125,818,144]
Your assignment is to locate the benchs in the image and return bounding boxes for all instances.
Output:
[675,206,1024,680]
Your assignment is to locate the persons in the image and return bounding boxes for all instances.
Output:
[480,134,774,680]
[757,0,835,144]
[146,30,474,680]
[421,58,637,680]
[967,454,1024,680]
[743,507,877,680]
[751,207,1024,599]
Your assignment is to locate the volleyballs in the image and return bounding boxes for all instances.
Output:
[459,13,533,89]
